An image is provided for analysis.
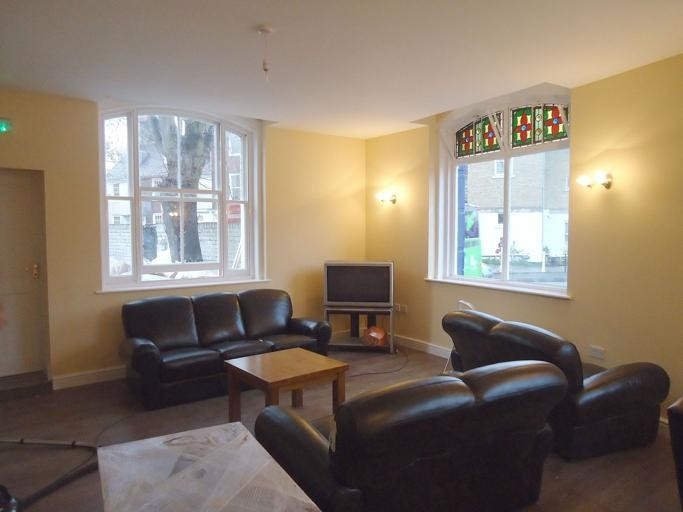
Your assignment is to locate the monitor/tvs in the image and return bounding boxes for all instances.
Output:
[322,260,395,307]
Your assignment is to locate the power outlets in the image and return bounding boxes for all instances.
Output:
[394,304,400,312]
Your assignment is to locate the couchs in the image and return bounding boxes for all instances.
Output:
[254,358,571,512]
[119,288,335,414]
[441,311,671,464]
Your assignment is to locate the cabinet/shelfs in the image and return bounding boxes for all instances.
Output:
[323,307,394,355]
